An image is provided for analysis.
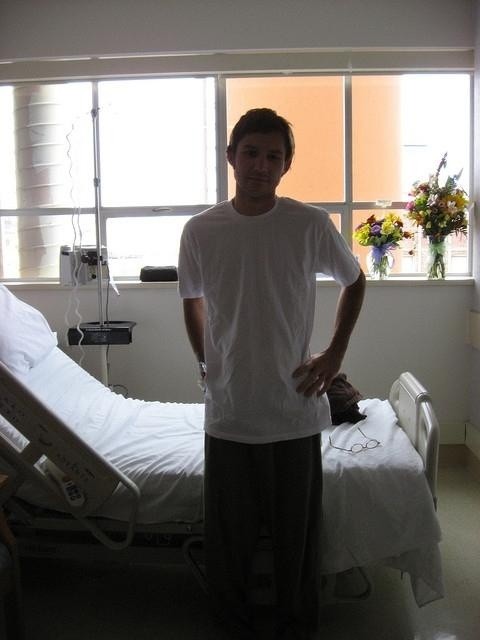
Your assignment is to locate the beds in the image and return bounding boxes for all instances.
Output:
[0,285,440,640]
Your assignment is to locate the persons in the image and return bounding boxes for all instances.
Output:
[178,109,366,640]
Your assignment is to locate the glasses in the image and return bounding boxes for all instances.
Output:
[328,427,379,453]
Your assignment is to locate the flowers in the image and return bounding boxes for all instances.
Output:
[403,152,471,279]
[352,213,414,279]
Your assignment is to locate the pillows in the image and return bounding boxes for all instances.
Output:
[1,283,60,374]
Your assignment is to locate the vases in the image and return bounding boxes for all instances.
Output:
[427,235,447,280]
[365,246,394,279]
